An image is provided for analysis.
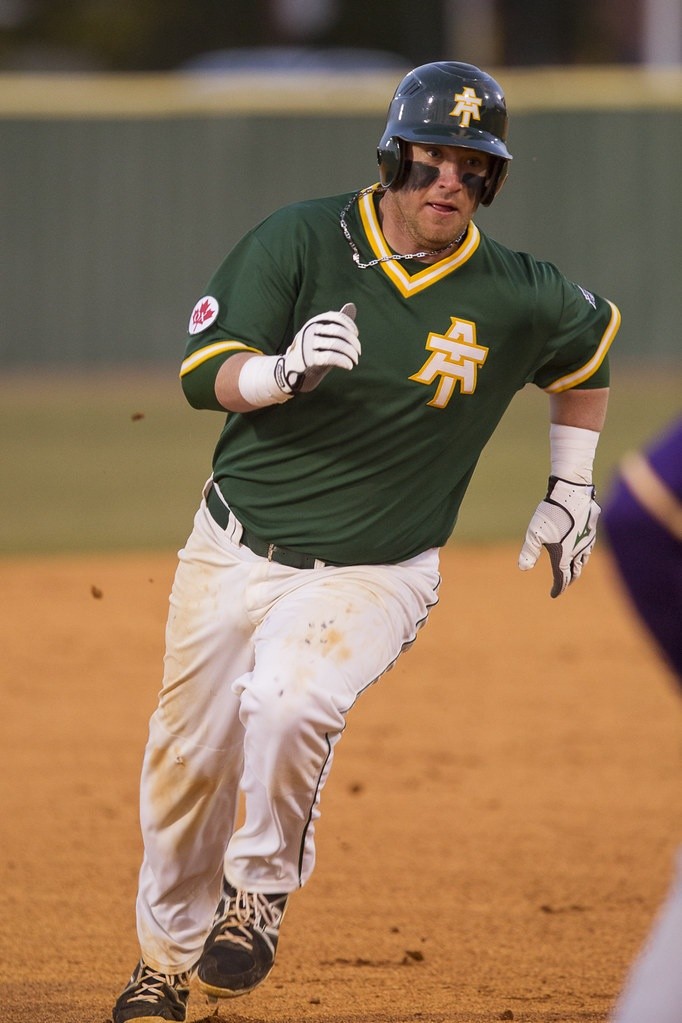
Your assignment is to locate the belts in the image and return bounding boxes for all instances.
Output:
[206,485,334,570]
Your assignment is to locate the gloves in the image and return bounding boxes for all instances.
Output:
[519,425,602,598]
[234,303,360,408]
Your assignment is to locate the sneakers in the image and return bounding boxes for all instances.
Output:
[113,955,199,1023]
[196,876,289,994]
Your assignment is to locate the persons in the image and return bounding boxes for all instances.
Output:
[114,62,620,1023]
[599,408,682,1023]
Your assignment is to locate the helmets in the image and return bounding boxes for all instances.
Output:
[378,60,511,207]
